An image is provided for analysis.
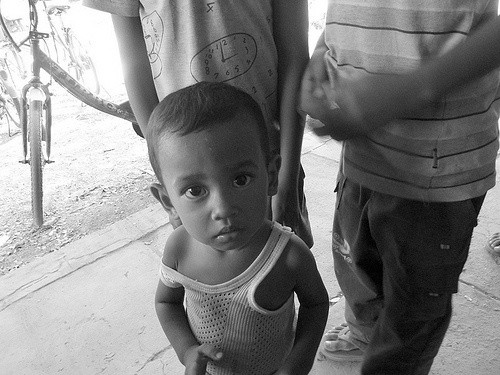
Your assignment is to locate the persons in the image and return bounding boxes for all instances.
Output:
[486,231,500,256]
[300,0,499,374]
[83,0,314,249]
[147,83,328,375]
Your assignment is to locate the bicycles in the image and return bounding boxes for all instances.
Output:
[0,0,145,225]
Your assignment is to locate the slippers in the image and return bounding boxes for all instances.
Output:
[320,322,367,362]
